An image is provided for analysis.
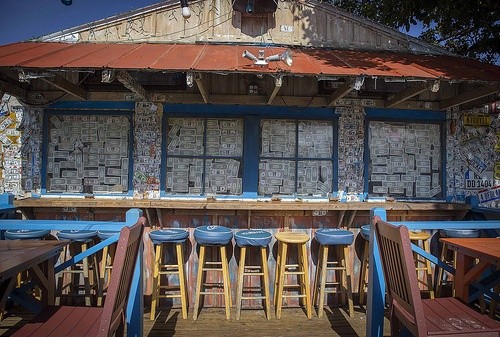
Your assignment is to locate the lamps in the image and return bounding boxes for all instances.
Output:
[242,49,293,66]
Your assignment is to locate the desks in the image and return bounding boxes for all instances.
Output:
[439,238,500,320]
[0,239,71,323]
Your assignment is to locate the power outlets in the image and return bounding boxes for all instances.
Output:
[62,207,78,213]
[312,210,327,217]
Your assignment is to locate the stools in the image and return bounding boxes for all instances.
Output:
[6,225,480,322]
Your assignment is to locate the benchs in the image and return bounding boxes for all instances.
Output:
[0,216,147,337]
[372,216,500,337]
